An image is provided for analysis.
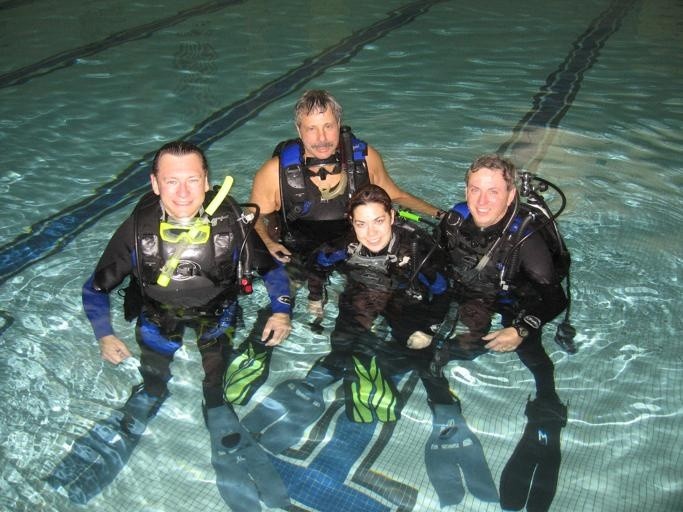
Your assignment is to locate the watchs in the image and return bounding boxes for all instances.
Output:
[513,324,530,337]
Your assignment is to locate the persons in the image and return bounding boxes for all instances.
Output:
[80,142,292,407]
[250,88,447,314]
[432,156,569,400]
[305,183,452,405]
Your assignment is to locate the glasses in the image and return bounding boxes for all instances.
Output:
[159,216,211,245]
